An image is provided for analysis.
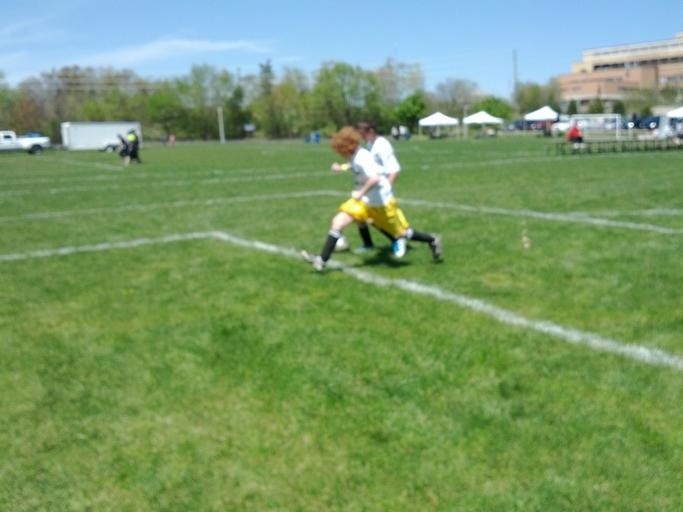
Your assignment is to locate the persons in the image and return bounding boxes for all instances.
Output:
[121,129,140,165]
[565,118,587,156]
[114,132,128,160]
[298,125,444,274]
[330,118,408,262]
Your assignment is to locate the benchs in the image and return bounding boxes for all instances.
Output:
[542,119,683,154]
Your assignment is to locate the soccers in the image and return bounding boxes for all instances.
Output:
[333,234,349,252]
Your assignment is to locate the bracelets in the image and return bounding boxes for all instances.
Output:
[340,163,348,171]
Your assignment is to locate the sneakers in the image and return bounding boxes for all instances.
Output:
[353,246,377,255]
[429,233,442,262]
[392,237,408,259]
[300,249,326,273]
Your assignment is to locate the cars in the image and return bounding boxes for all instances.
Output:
[508,114,682,135]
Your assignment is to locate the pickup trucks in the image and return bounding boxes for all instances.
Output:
[1,129,51,155]
